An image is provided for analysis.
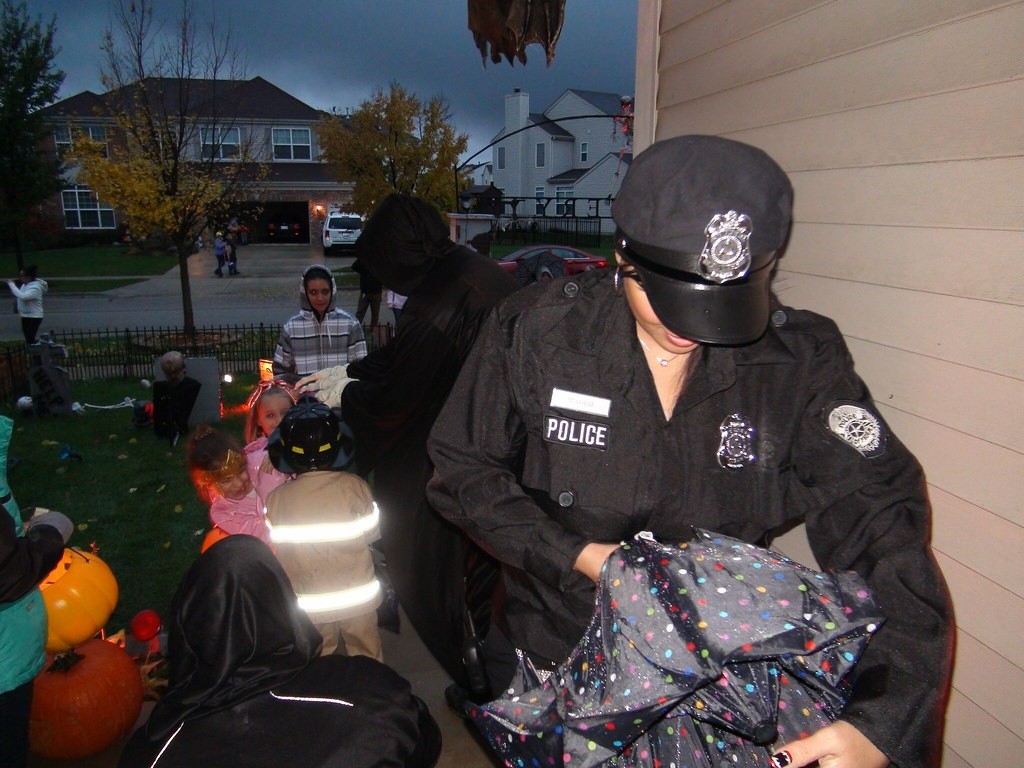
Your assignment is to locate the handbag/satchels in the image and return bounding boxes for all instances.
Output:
[13,283,23,314]
[225,232,233,240]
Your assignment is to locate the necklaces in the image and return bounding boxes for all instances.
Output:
[638,338,679,367]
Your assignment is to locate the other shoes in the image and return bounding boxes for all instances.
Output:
[215,269,223,277]
[229,270,240,275]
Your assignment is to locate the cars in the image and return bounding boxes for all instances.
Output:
[494,245,609,283]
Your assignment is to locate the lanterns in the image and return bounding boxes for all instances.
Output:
[28,639,143,759]
[38,547,120,656]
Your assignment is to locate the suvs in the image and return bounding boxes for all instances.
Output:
[322,212,364,250]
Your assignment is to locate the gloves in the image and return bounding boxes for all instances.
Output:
[291,359,354,394]
[311,378,358,413]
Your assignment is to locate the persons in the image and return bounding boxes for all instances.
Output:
[242,380,298,499]
[213,218,251,277]
[191,423,274,554]
[7,263,49,342]
[118,533,444,767]
[387,288,408,328]
[426,133,957,768]
[293,192,538,722]
[264,398,386,667]
[272,264,368,387]
[351,257,383,339]
[0,413,75,768]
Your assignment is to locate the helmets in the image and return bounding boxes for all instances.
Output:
[216,231,223,237]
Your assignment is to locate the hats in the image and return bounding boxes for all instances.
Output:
[609,133,793,348]
[265,396,360,476]
